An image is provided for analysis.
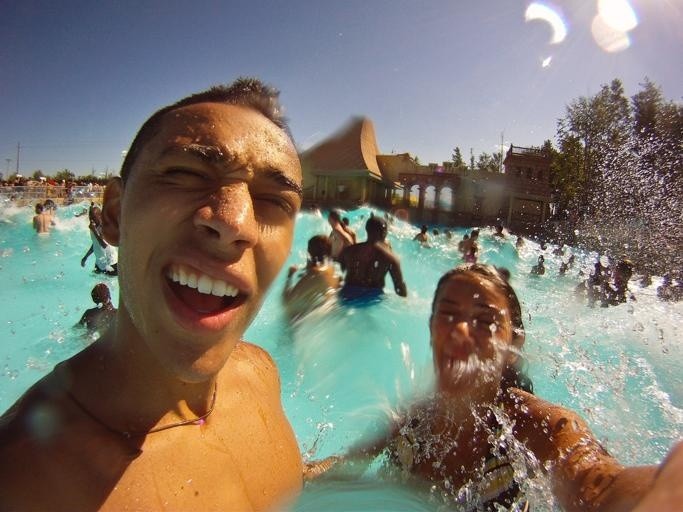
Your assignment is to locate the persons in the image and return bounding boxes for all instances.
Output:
[0,74,305,512]
[302,260,682,510]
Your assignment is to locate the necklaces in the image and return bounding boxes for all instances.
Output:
[53,360,219,441]
